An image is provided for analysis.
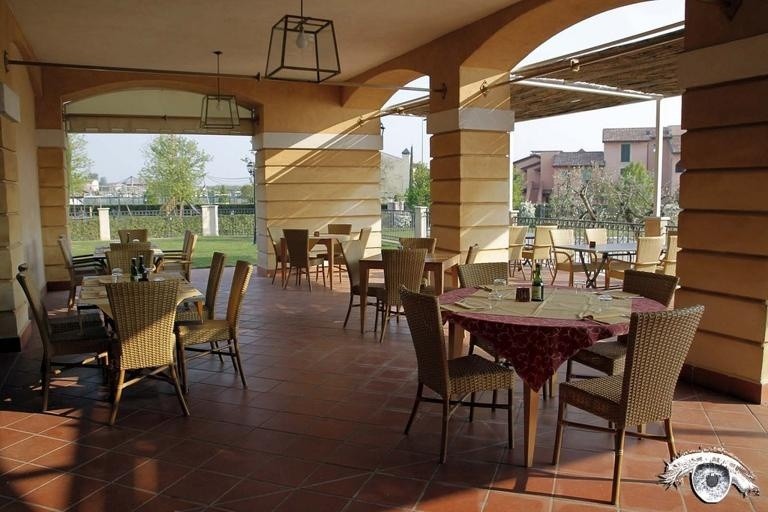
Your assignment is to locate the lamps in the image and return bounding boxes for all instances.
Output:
[197,49,243,130]
[263,0,343,87]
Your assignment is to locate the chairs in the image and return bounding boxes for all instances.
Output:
[11,221,255,430]
[453,258,547,413]
[392,283,519,464]
[264,217,682,344]
[549,303,706,505]
[561,267,680,385]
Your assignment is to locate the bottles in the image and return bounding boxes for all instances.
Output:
[531,261,544,300]
[110,255,166,286]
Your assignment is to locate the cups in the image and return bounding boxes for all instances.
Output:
[492,278,507,299]
[574,280,586,295]
